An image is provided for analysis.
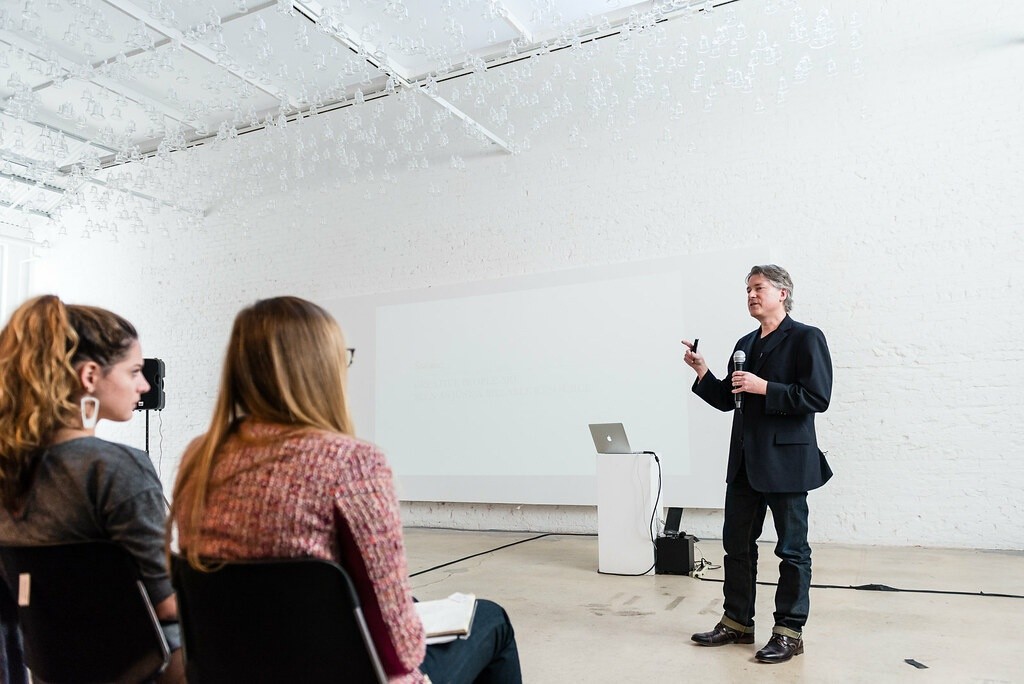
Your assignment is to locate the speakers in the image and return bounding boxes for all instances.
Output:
[656,535,695,576]
[133,357,166,412]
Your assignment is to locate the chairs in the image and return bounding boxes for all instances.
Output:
[168,554,389,684]
[1,541,186,684]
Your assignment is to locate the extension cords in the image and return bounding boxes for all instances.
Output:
[693,565,709,577]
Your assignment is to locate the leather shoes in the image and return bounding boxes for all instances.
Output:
[756,634,805,663]
[690,621,756,647]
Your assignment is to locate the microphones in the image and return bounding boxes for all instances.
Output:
[734,351,745,408]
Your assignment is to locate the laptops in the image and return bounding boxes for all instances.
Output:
[589,423,653,455]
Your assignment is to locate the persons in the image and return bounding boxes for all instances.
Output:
[681,265,833,664]
[0,295,183,684]
[165,297,523,684]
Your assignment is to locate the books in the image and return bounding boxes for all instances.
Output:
[413,593,476,645]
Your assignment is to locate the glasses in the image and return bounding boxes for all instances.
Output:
[346,347,355,368]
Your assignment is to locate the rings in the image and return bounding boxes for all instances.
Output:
[737,382,738,385]
